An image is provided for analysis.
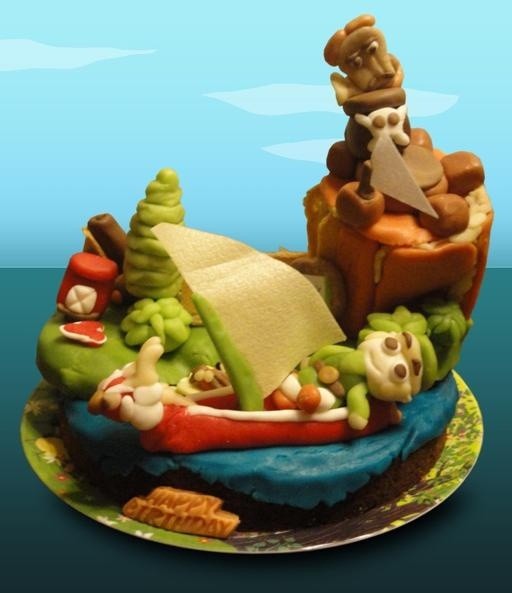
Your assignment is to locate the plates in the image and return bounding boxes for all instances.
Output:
[20,367,486,556]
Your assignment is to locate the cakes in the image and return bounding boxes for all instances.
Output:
[35,9,494,537]
